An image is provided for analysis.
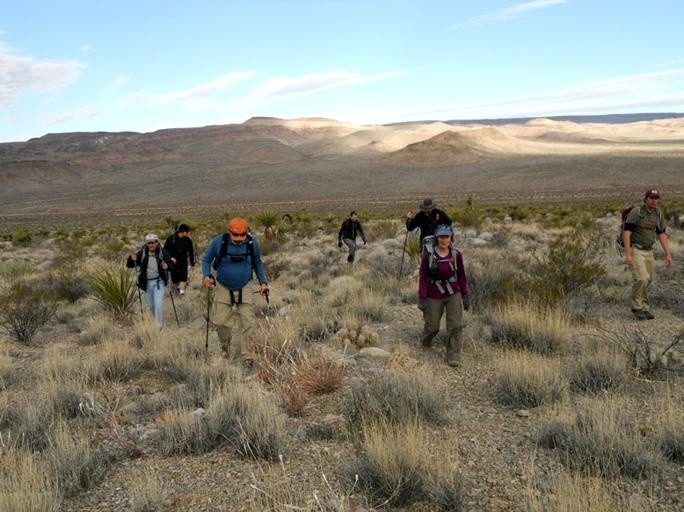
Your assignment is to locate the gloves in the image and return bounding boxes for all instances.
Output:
[417,298,430,314]
[461,296,471,311]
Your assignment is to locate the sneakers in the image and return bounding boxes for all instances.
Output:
[641,309,655,319]
[218,341,232,359]
[443,354,459,367]
[631,306,647,320]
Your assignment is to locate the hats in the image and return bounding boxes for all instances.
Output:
[419,198,437,209]
[227,217,249,241]
[433,224,454,236]
[645,188,661,198]
[144,234,159,242]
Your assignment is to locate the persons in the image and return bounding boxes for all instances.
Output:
[622,190,674,319]
[127,233,176,327]
[417,224,470,366]
[163,223,195,296]
[338,211,367,262]
[202,218,268,365]
[406,199,453,251]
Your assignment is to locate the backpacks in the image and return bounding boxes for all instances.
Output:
[420,234,458,282]
[617,205,660,247]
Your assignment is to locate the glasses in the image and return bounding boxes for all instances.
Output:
[227,229,249,237]
